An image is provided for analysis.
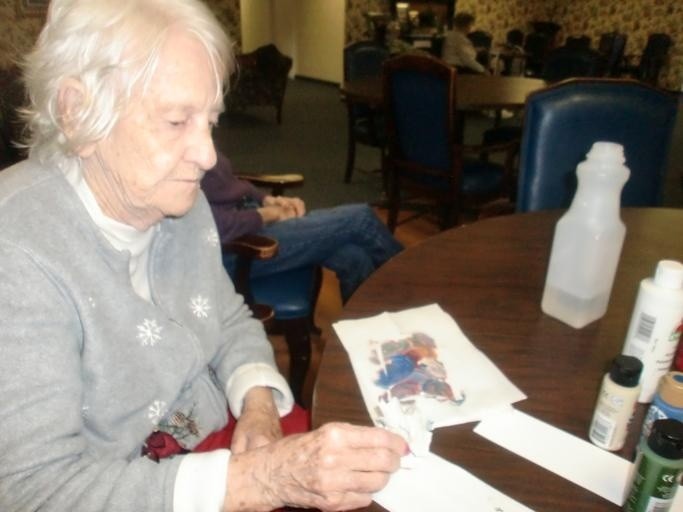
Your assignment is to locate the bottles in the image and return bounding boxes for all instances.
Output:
[539,139,632,329]
[588,258,683,512]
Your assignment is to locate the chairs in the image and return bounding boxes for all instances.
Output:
[217,169,325,405]
[342,21,683,236]
[215,44,294,124]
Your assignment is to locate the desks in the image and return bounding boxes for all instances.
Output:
[309,205,683,512]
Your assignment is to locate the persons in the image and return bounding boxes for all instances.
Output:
[441,10,514,121]
[197,146,405,308]
[488,27,526,77]
[1,1,412,511]
